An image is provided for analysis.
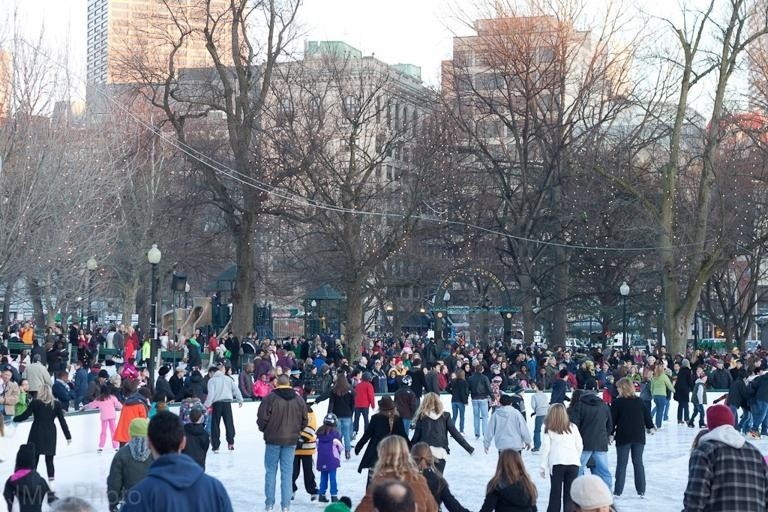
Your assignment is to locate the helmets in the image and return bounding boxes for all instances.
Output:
[323,412,340,427]
[401,374,413,387]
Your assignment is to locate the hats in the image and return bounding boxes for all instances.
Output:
[681,358,691,368]
[583,378,598,391]
[98,369,110,378]
[158,365,170,376]
[378,395,398,409]
[569,471,614,511]
[353,350,423,367]
[129,416,148,440]
[14,442,37,472]
[706,404,736,431]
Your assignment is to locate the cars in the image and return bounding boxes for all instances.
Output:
[687,337,763,349]
[511,327,590,351]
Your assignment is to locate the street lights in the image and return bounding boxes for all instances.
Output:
[184,281,191,310]
[442,289,451,343]
[310,300,317,337]
[619,281,630,353]
[170,260,187,374]
[145,242,163,395]
[85,256,97,334]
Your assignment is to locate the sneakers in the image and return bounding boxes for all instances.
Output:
[459,431,468,438]
[228,443,235,451]
[97,448,104,454]
[663,418,673,424]
[48,477,56,484]
[737,427,768,440]
[331,495,340,503]
[290,492,296,501]
[310,495,318,504]
[111,447,120,453]
[530,447,540,453]
[677,420,706,428]
[318,494,330,503]
[66,438,73,446]
[213,448,220,453]
[345,449,351,461]
[656,425,665,432]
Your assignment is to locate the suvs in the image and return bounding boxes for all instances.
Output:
[631,338,658,355]
